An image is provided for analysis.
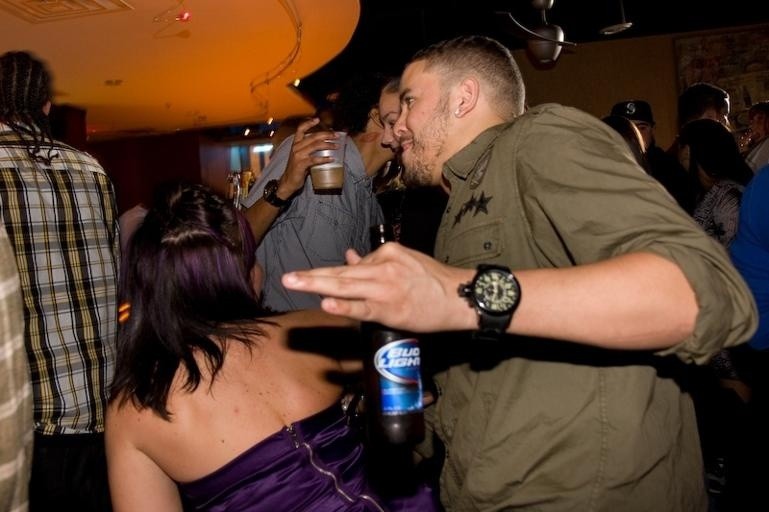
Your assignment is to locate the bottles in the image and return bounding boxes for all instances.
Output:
[360,221,427,449]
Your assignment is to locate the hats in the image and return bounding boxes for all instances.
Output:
[611,100,655,125]
[681,119,755,186]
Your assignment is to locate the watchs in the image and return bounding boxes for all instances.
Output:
[263,180,288,207]
[459,263,522,337]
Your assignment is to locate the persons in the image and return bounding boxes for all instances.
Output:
[282,35,758,512]
[240,76,401,312]
[599,84,769,512]
[105,183,444,512]
[0,51,155,512]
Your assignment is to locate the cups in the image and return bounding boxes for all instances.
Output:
[302,130,349,192]
